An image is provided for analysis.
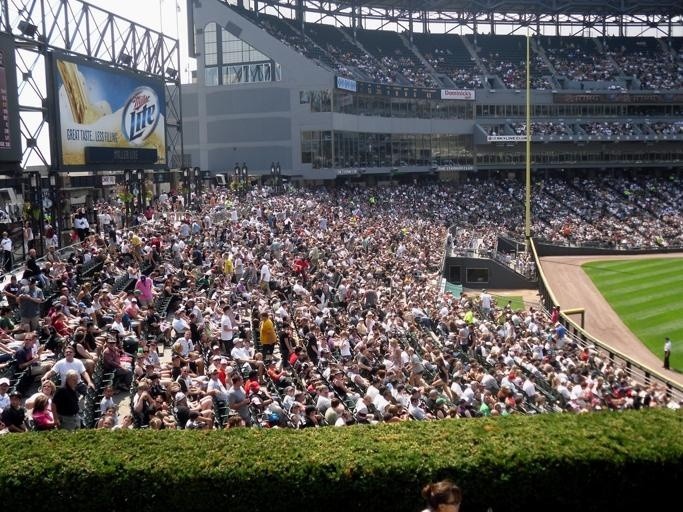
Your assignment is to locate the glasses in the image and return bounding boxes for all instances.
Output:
[448,499,462,507]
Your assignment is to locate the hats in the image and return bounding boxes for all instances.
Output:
[0,260,674,426]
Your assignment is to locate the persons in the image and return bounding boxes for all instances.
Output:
[420,478,461,512]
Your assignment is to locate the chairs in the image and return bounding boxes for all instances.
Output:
[1,245,176,428]
